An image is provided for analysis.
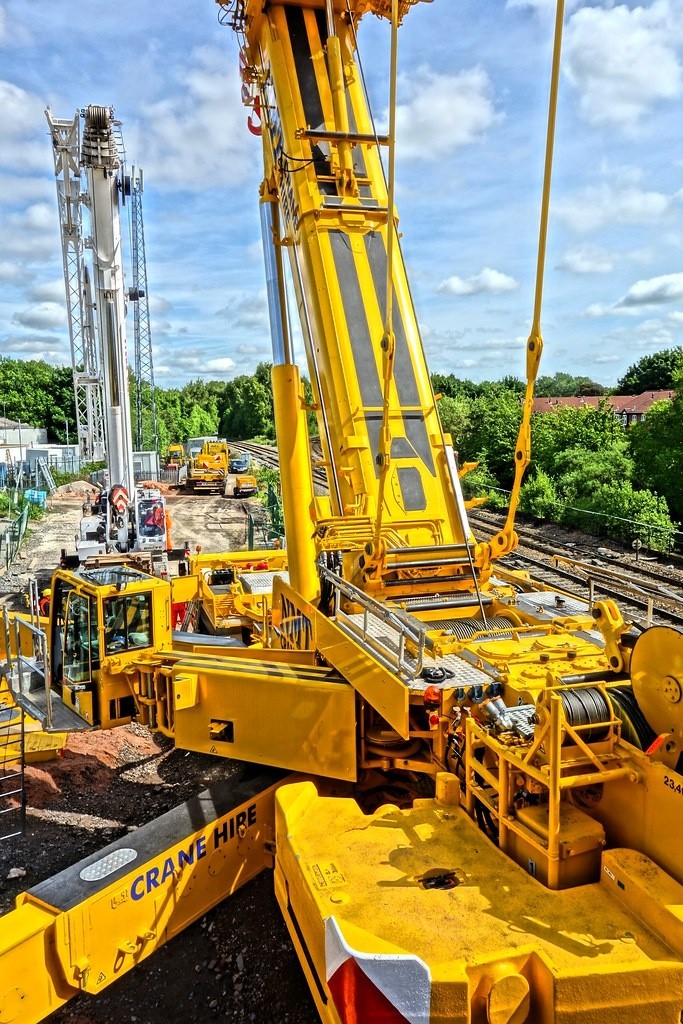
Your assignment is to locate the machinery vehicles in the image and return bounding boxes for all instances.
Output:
[0,0,683,1024]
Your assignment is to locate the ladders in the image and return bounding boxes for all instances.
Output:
[0,658,27,846]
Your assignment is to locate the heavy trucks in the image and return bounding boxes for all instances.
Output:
[187,436,218,460]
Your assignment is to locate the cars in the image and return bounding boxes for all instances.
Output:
[229,460,247,473]
